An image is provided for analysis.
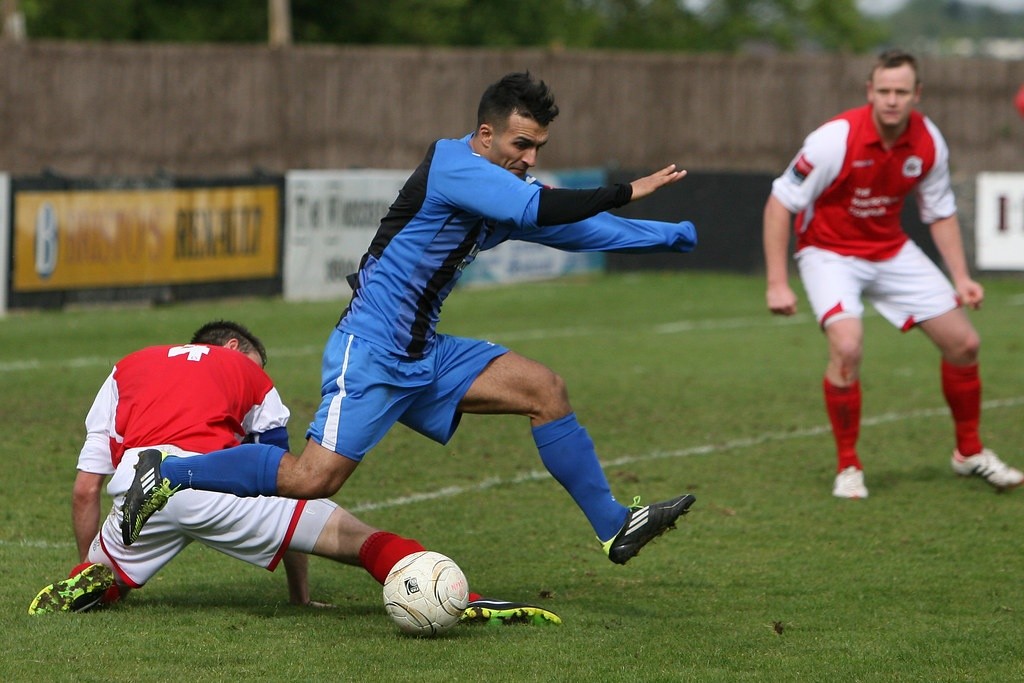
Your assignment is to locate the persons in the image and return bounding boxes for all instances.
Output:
[116,69,693,567]
[761,48,1023,497]
[25,321,565,627]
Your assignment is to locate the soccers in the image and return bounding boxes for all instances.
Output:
[382,551,469,637]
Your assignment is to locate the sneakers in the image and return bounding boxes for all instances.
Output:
[28,564,114,617]
[119,450,179,546]
[596,495,696,565]
[833,465,867,498]
[460,597,561,626]
[952,448,1024,491]
